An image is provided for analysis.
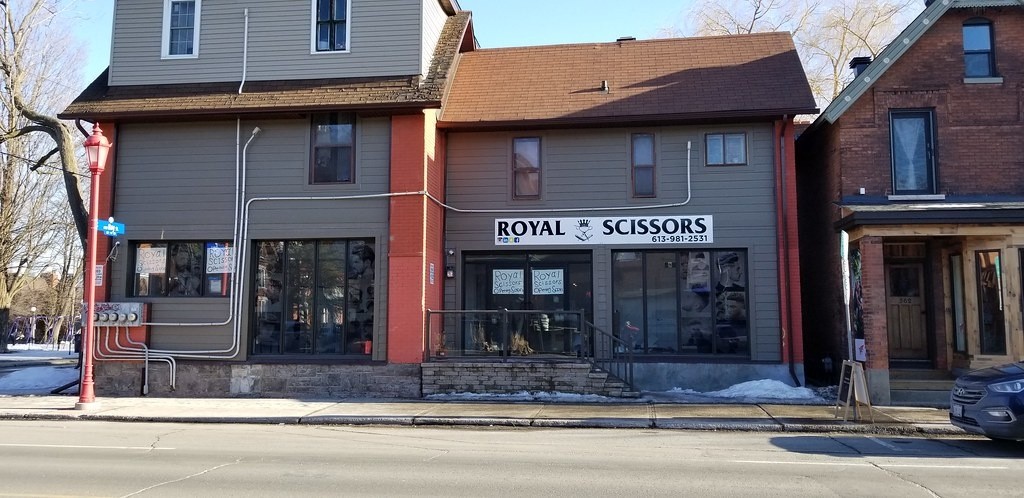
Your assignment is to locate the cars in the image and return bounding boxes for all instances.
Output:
[949,361,1023,443]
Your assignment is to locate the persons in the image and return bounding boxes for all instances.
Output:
[692,253,708,269]
[682,282,710,315]
[715,291,746,321]
[682,320,712,353]
[717,253,746,291]
[168,244,200,297]
[348,284,374,342]
[257,278,282,313]
[254,314,281,353]
[351,244,375,280]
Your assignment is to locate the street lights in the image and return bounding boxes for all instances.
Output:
[31,307,37,346]
[75,122,114,411]
